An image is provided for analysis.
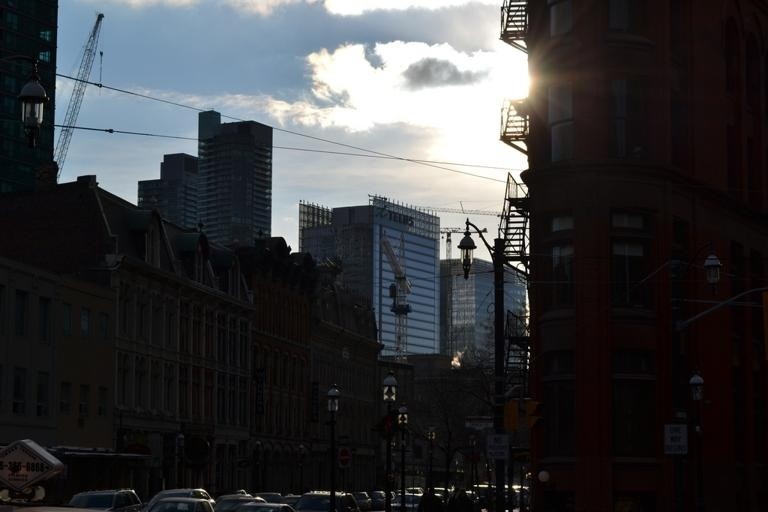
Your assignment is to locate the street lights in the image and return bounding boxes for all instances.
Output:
[176,432,185,489]
[298,443,304,492]
[326,382,343,511]
[254,440,262,493]
[460,218,506,510]
[427,430,437,495]
[671,244,724,510]
[382,370,409,512]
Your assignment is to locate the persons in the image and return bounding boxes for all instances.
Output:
[415,485,481,511]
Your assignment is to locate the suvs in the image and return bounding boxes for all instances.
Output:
[65,488,144,511]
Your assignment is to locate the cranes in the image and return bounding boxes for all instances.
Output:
[440,226,487,258]
[54,12,104,182]
[379,229,412,365]
